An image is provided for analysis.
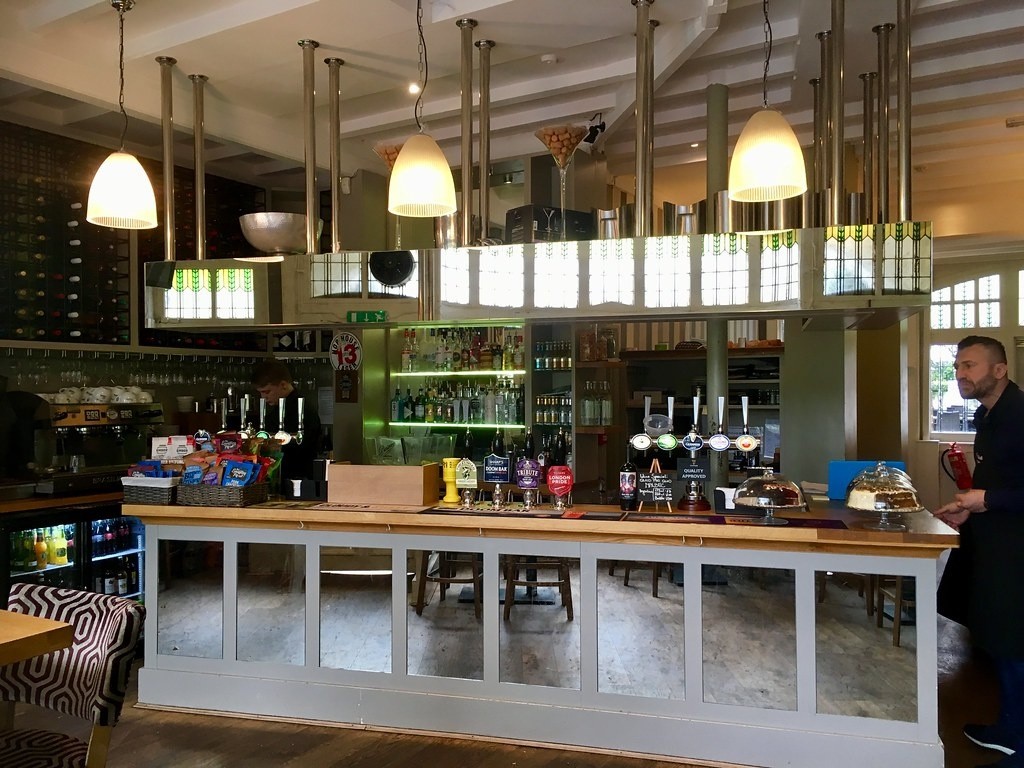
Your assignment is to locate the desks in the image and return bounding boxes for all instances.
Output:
[456,557,556,607]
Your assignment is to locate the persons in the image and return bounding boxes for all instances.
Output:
[252,359,321,503]
[933,336,1024,755]
[620,474,636,495]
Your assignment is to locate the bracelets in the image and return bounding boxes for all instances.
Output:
[983,492,989,510]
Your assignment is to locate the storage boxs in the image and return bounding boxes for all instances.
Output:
[327,461,439,506]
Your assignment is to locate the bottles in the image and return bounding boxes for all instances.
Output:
[395,326,525,373]
[618,447,638,511]
[535,340,572,369]
[580,380,614,426]
[11,518,140,594]
[464,426,572,483]
[535,397,572,423]
[388,376,524,424]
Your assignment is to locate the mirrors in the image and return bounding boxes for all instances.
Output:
[143,525,939,748]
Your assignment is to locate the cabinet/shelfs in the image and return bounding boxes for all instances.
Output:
[387,320,527,429]
[619,347,784,474]
[0,499,143,607]
[527,319,626,484]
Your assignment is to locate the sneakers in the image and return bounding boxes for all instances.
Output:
[963,724,1016,756]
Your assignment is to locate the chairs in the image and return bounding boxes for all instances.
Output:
[0,582,148,768]
[415,550,574,623]
[876,575,916,647]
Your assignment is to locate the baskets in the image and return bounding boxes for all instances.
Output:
[123,483,177,506]
[176,481,271,507]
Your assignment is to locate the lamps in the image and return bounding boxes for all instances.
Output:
[727,0,808,204]
[87,0,164,230]
[385,0,458,219]
